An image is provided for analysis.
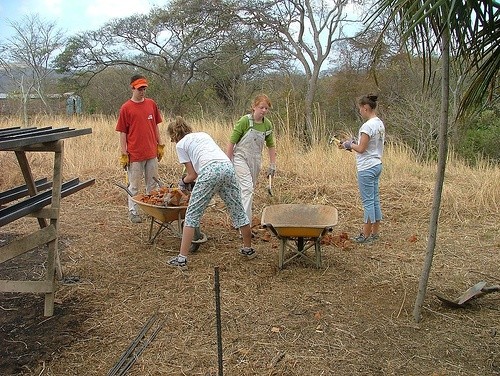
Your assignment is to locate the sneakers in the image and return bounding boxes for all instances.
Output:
[165,256,187,268]
[239,248,256,260]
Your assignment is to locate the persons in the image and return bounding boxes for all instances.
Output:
[227,94,276,239]
[116,75,165,223]
[343,93,385,242]
[167,116,256,266]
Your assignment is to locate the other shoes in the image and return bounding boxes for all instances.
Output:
[127,212,142,222]
[352,235,381,244]
[239,231,255,240]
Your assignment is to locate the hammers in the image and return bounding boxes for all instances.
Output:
[268,173,272,197]
[126,166,131,187]
[330,135,352,152]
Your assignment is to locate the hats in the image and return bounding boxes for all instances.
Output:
[130,79,148,90]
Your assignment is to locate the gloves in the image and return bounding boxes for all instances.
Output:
[157,144,165,162]
[266,167,275,179]
[179,177,186,187]
[121,152,129,170]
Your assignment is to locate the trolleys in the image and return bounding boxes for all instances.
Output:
[258,203,338,269]
[115,175,218,254]
